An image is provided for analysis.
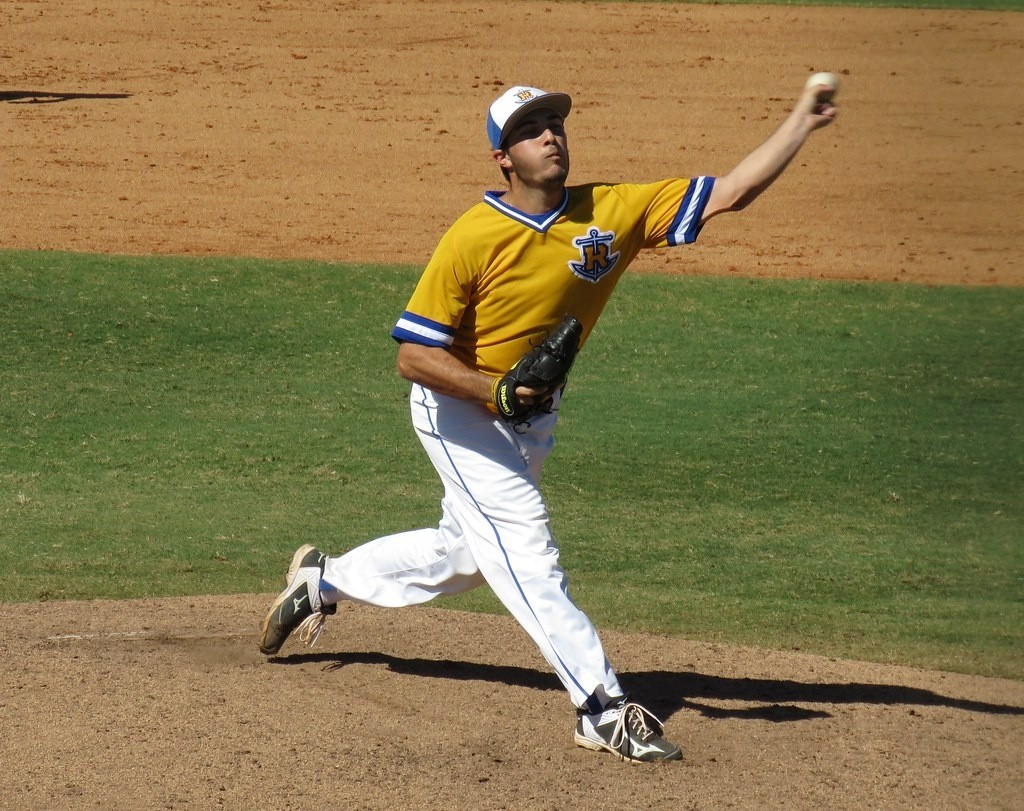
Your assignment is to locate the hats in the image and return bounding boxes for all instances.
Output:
[487,85,571,149]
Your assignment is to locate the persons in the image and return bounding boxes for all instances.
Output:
[259,70,838,766]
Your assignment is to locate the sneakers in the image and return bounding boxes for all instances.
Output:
[259,544,337,655]
[574,692,683,766]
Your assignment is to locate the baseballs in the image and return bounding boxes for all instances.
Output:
[800,70,839,85]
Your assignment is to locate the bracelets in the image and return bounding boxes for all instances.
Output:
[491,376,503,407]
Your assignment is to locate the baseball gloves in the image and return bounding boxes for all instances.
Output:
[492,312,586,426]
